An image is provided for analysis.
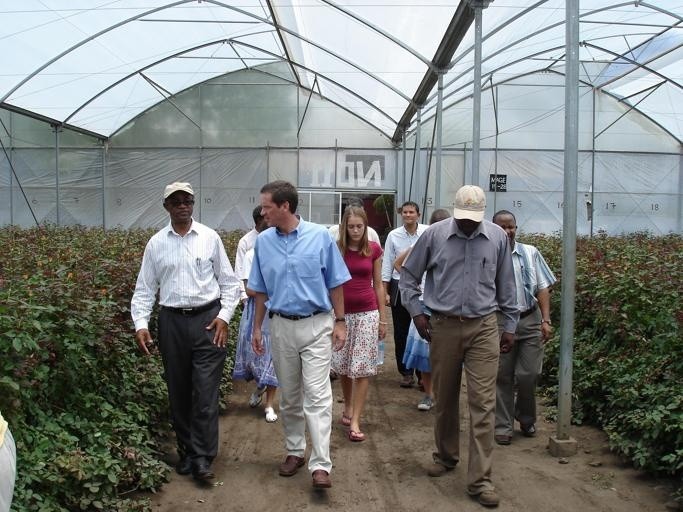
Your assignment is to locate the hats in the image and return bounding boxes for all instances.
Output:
[163,181,194,201]
[453,185,486,222]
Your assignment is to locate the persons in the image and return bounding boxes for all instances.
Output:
[230,194,451,442]
[489,209,558,447]
[395,183,521,508]
[128,180,242,484]
[243,178,354,489]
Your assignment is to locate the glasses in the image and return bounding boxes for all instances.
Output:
[170,200,194,207]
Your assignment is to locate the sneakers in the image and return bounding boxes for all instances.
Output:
[418,397,433,410]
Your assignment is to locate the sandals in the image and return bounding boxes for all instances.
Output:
[264,407,278,422]
[249,386,267,408]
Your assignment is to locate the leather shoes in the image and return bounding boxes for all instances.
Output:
[428,463,445,476]
[495,435,510,445]
[473,489,499,505]
[176,455,193,474]
[193,465,216,480]
[400,374,414,386]
[312,470,331,488]
[520,424,535,434]
[417,376,423,385]
[279,455,305,475]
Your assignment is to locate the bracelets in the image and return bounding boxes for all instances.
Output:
[539,319,551,325]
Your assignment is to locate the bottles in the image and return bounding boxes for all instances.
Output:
[378,339,385,365]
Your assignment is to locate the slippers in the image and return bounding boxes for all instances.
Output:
[348,430,365,441]
[342,410,351,425]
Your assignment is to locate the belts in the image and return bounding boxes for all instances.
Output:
[162,301,219,315]
[278,311,320,321]
[447,316,473,322]
[519,305,537,319]
